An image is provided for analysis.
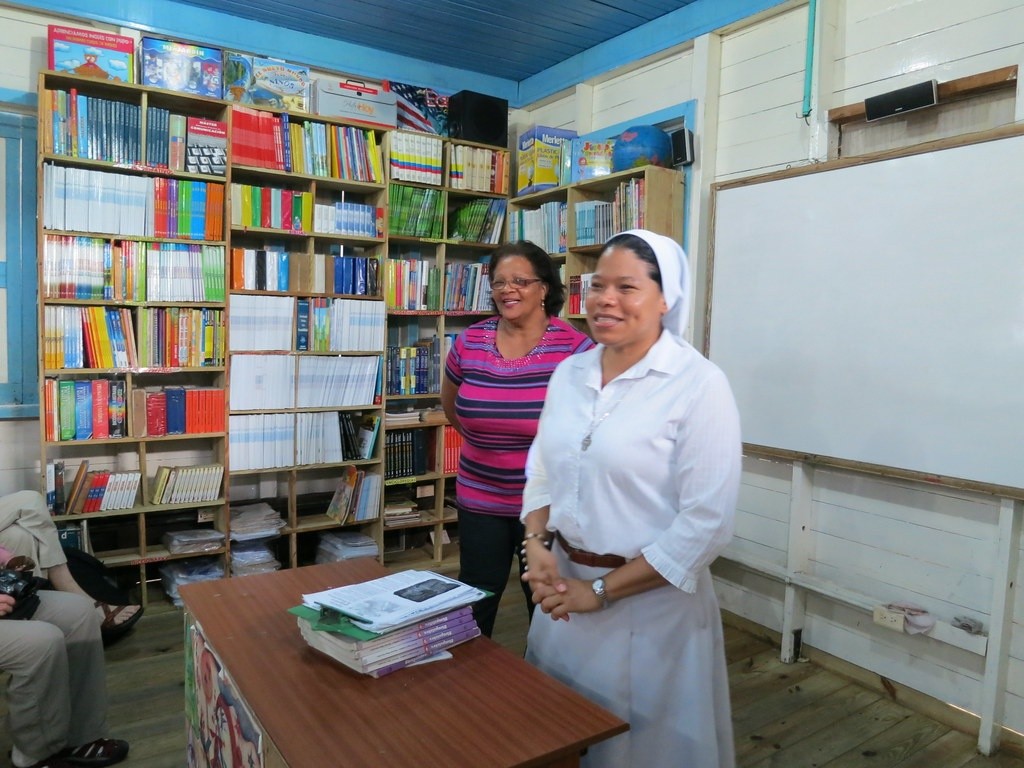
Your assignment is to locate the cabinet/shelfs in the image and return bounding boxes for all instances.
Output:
[34,68,689,612]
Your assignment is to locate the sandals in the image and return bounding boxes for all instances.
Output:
[6,736,130,768]
[93,598,144,633]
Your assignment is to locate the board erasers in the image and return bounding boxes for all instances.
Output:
[951,615,983,634]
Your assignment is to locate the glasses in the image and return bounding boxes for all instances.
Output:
[490,278,542,290]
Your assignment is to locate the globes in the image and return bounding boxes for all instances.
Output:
[612,125,673,172]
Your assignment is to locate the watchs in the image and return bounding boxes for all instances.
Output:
[592,577,610,610]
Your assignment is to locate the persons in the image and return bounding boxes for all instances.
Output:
[441,240,599,637]
[528,230,742,768]
[161,528,227,609]
[0,490,143,636]
[0,579,127,768]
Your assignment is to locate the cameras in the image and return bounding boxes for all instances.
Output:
[0,571,30,598]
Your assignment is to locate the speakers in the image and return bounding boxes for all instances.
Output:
[864,79,939,122]
[447,90,508,148]
[671,128,695,166]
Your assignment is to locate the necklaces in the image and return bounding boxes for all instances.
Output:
[581,382,634,451]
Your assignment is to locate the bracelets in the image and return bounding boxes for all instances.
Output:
[521,534,548,570]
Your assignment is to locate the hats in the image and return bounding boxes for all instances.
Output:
[605,229,690,337]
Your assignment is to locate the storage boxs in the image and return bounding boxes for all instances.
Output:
[558,139,571,187]
[516,124,577,198]
[57,525,80,550]
[47,24,398,129]
[571,138,615,182]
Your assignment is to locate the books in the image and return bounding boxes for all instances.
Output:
[46,459,225,515]
[508,168,651,317]
[386,127,508,532]
[44,87,186,172]
[42,160,223,242]
[44,378,226,442]
[286,568,494,679]
[230,103,383,577]
[41,233,227,302]
[42,305,225,369]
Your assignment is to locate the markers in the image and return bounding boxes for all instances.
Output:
[881,604,928,614]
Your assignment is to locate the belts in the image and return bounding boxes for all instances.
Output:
[555,530,625,568]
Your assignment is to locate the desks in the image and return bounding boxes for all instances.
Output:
[172,554,630,768]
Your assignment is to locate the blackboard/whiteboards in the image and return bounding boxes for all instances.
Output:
[700,120,1024,503]
